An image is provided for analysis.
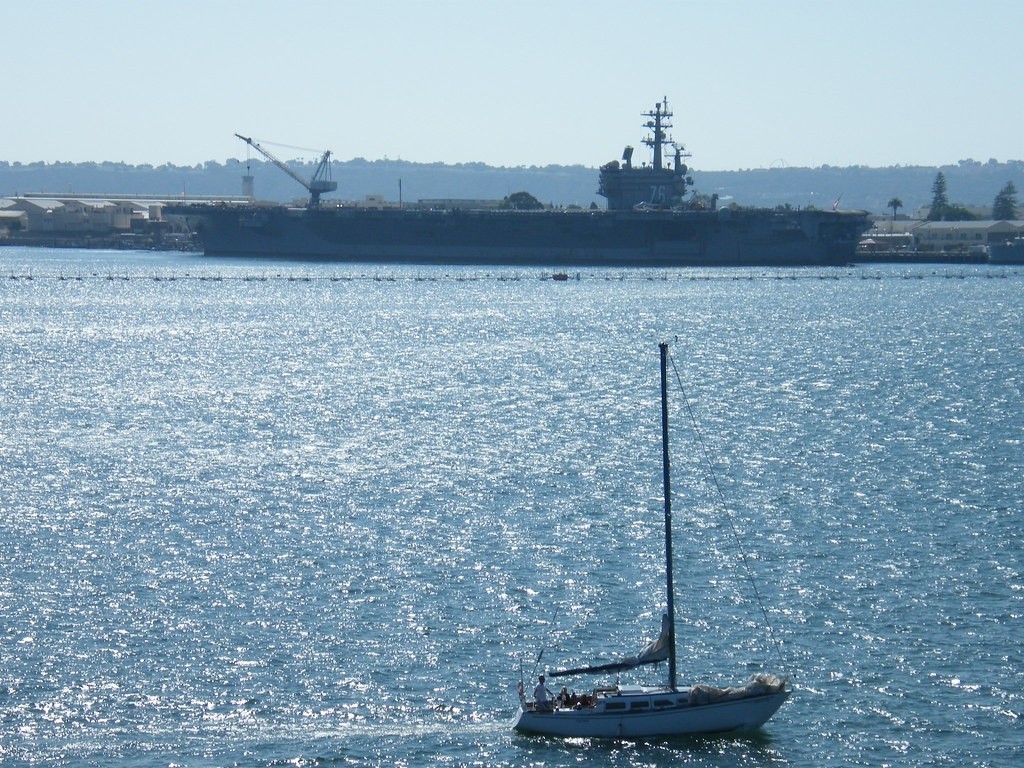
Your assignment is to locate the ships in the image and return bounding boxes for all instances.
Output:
[198,96,876,266]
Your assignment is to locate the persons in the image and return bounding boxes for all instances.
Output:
[557,686,572,707]
[532,675,556,709]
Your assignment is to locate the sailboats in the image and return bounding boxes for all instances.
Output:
[511,341,797,738]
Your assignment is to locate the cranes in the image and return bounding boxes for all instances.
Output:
[235,136,338,209]
